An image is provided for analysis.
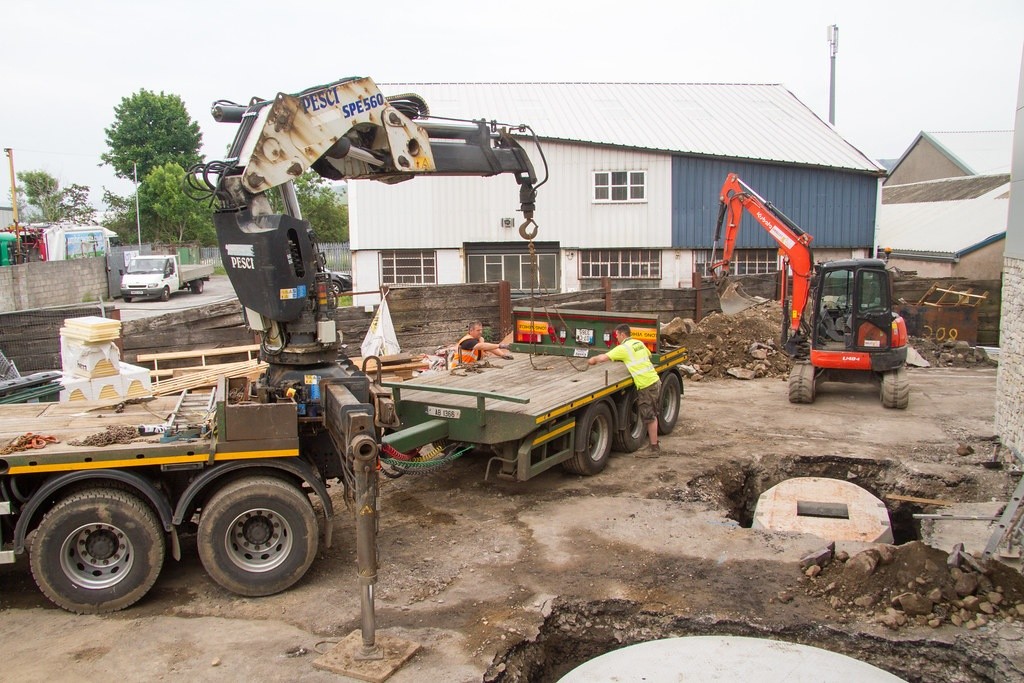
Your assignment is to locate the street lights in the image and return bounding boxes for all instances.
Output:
[106,155,143,256]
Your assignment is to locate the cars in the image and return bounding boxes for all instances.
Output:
[321,267,353,294]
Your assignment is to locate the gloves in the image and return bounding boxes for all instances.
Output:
[499,344,510,349]
[502,354,514,360]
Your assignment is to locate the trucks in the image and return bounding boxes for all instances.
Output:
[119,255,216,303]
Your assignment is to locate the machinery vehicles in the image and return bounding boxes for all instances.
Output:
[0,147,29,266]
[707,172,909,410]
[0,75,549,615]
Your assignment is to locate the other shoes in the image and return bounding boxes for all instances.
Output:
[633,440,661,458]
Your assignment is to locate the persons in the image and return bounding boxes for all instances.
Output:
[588,323,663,459]
[451,319,516,368]
